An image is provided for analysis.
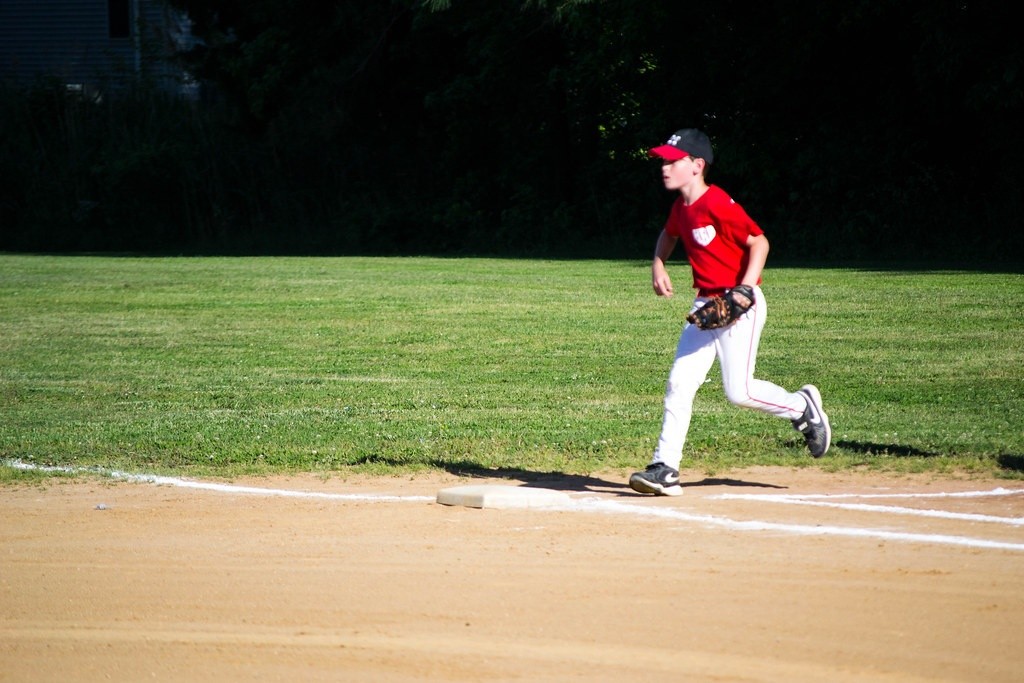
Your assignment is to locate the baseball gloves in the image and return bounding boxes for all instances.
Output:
[686,285,756,330]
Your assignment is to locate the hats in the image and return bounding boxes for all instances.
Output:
[650,128,713,164]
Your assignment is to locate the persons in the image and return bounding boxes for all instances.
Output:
[630,130,831,496]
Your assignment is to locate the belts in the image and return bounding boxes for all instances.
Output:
[698,289,730,298]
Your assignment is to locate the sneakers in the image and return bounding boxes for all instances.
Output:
[630,462,682,496]
[791,384,831,458]
